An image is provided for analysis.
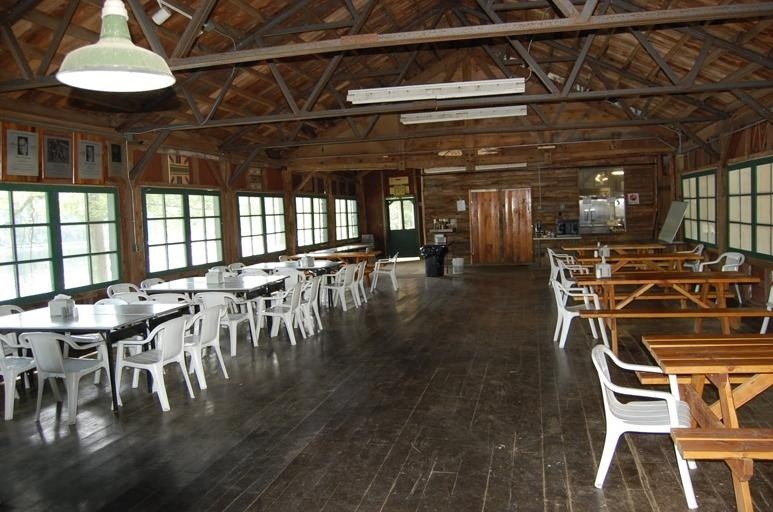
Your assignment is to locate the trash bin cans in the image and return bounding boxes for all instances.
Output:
[423,245,446,277]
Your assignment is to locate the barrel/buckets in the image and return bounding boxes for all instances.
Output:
[453,258,464,274]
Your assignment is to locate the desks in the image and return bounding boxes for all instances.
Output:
[309,243,375,263]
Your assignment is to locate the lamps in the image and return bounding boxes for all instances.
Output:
[55,0,177,93]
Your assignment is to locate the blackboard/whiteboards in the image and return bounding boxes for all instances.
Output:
[658,201,689,244]
